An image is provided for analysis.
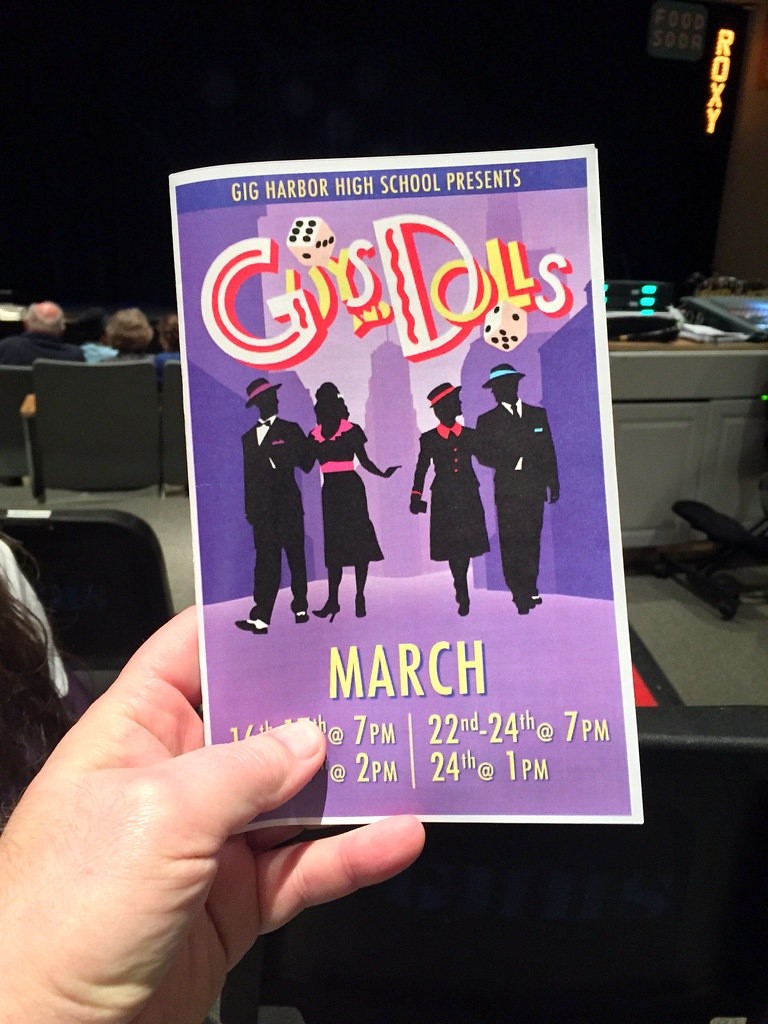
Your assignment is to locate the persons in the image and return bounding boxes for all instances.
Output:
[0,300,181,408]
[0,535,426,1024]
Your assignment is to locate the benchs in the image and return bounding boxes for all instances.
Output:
[71,668,768,1024]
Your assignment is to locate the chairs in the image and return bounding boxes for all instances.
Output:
[0,509,174,670]
[159,359,191,498]
[20,358,161,505]
[0,364,34,481]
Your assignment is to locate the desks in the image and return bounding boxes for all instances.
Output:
[609,351,768,550]
